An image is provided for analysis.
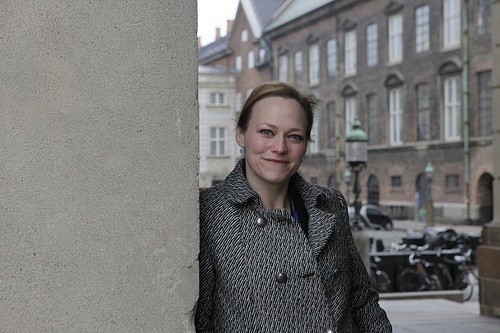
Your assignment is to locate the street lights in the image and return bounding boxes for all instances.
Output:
[344,120,369,228]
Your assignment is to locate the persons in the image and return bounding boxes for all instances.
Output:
[195,80,394,333]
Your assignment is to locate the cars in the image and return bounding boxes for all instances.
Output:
[346,205,393,231]
